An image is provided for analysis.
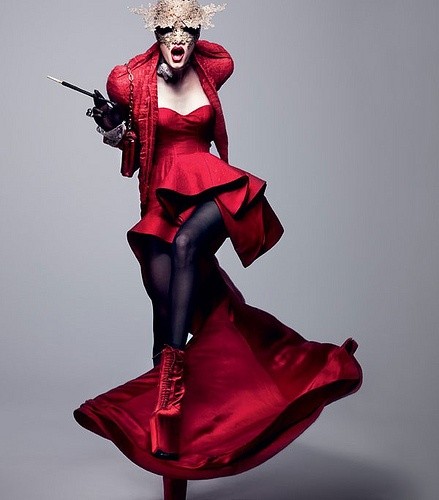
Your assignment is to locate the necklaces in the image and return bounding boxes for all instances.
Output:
[157,56,191,81]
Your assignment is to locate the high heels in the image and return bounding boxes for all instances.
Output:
[149,345,188,461]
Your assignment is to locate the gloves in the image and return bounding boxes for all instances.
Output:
[90,89,125,132]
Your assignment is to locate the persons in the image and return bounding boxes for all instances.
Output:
[86,0,285,500]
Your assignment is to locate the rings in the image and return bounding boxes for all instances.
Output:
[86,108,94,117]
